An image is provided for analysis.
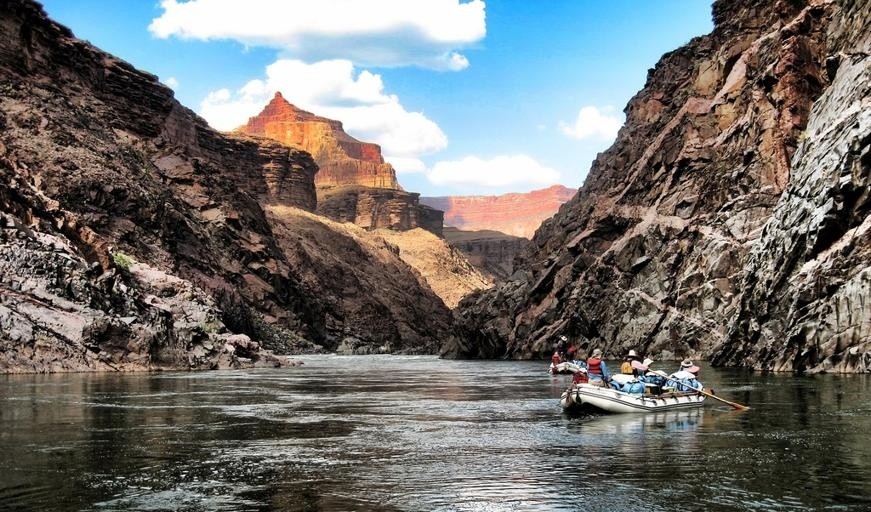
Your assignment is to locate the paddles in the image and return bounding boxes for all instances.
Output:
[648,368,748,410]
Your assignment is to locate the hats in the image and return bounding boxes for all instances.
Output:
[643,358,655,367]
[684,365,701,373]
[591,348,603,359]
[681,358,694,367]
[625,349,640,357]
[561,335,568,342]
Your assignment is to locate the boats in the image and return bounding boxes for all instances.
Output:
[571,405,703,439]
[554,372,710,417]
[546,360,589,377]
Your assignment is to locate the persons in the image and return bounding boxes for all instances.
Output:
[679,358,699,379]
[552,343,578,365]
[556,335,568,362]
[621,349,651,376]
[586,348,613,387]
[573,368,588,383]
